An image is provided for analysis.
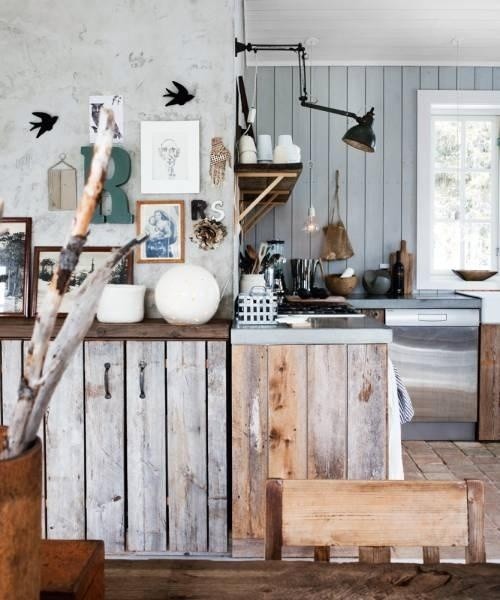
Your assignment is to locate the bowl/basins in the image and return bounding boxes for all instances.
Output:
[90,282,148,326]
[325,270,360,297]
[274,145,302,165]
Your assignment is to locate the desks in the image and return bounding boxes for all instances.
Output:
[101,558,500,600]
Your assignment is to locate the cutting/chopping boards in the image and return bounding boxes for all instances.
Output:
[287,293,347,306]
[389,240,415,295]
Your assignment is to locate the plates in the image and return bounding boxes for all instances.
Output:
[452,268,498,284]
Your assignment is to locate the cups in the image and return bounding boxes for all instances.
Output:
[277,134,293,147]
[241,151,259,165]
[255,134,274,161]
[240,134,258,155]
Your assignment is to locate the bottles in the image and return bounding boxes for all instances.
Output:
[391,251,405,298]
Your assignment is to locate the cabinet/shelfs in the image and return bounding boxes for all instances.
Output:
[235,162,304,235]
[1,337,231,558]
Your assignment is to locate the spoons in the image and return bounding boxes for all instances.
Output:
[245,244,259,273]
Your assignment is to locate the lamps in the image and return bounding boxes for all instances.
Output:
[301,36,321,236]
[235,38,377,153]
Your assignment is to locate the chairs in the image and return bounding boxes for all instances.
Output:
[266,477,486,565]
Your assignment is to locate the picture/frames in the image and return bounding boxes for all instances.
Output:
[30,246,134,324]
[139,120,201,195]
[135,200,185,264]
[0,216,32,320]
